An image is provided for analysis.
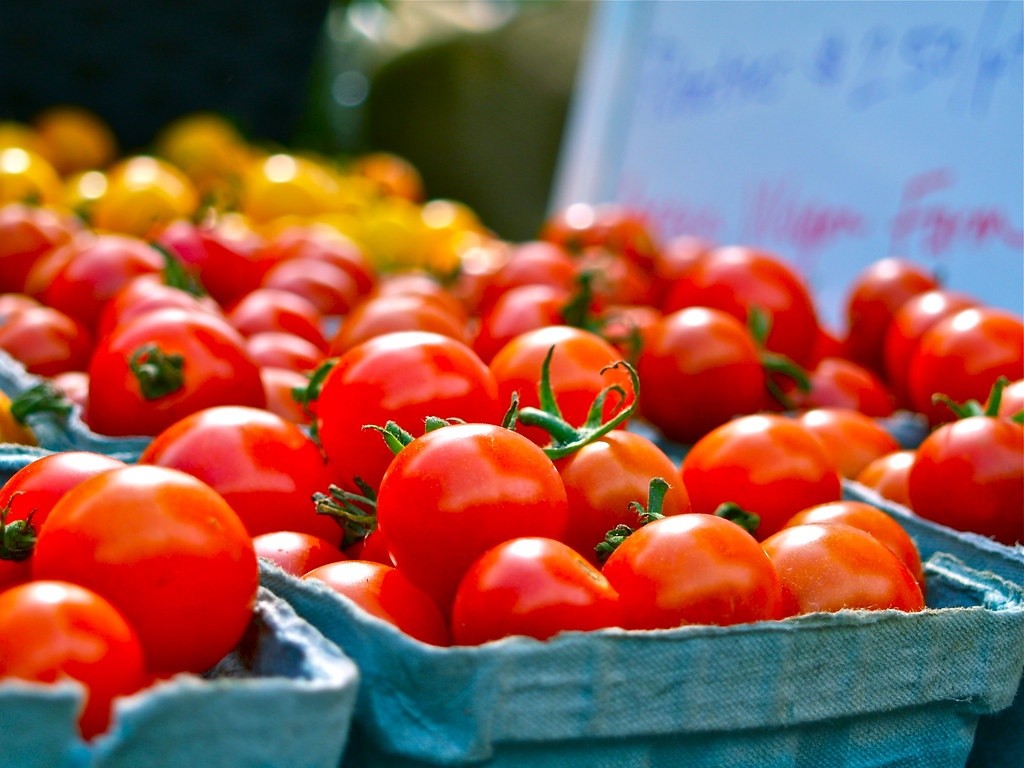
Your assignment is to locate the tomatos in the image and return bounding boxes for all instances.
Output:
[2,111,1024,747]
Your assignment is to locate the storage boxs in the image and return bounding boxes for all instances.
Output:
[0,446,1024,768]
